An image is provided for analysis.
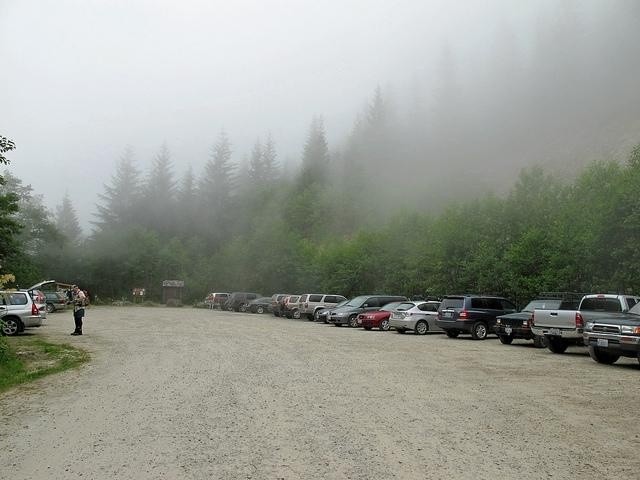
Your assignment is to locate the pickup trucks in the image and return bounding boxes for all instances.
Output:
[529,293,640,354]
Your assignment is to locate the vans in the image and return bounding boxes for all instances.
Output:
[325,295,408,328]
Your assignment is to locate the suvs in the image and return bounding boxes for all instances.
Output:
[9,287,67,320]
[296,294,349,321]
[0,285,55,336]
[583,302,640,366]
[433,295,518,340]
[206,289,304,319]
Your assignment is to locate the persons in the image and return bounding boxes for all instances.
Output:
[67,285,86,335]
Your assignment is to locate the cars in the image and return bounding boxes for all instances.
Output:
[355,301,405,331]
[388,301,445,335]
[494,299,581,349]
[316,300,349,324]
